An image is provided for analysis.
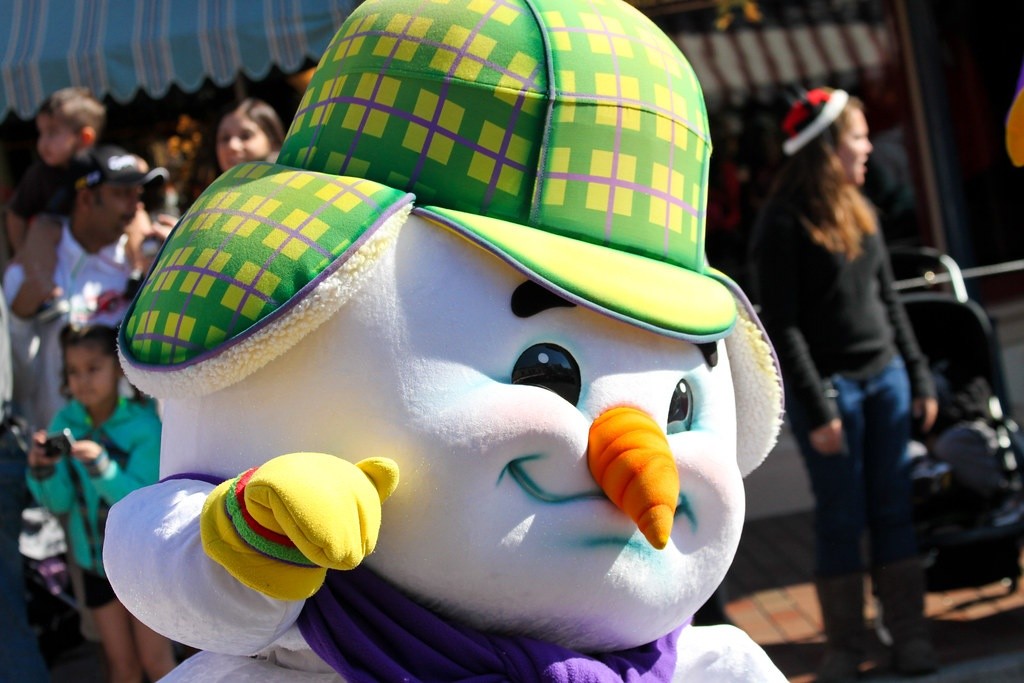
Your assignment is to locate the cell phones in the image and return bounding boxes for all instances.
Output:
[42,429,75,455]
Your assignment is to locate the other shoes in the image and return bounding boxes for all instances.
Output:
[816,641,862,683]
[891,637,938,678]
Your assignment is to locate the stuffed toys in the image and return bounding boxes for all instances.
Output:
[102,1,787,683]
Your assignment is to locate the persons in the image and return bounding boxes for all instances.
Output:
[753,85,939,683]
[0,86,288,683]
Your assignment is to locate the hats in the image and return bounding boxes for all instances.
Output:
[783,86,847,154]
[74,147,169,191]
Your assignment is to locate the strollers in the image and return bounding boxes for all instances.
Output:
[891,246,1024,637]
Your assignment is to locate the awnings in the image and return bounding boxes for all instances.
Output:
[0,0,360,121]
[671,23,896,109]
